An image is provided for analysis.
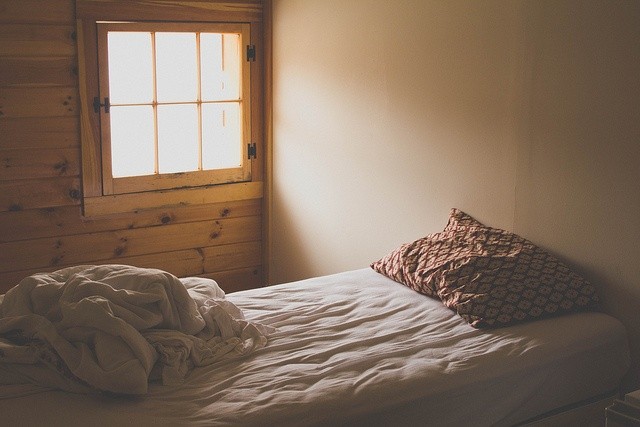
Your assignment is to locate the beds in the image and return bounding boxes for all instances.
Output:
[1,266,630,425]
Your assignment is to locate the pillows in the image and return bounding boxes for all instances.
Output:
[370,207,600,330]
